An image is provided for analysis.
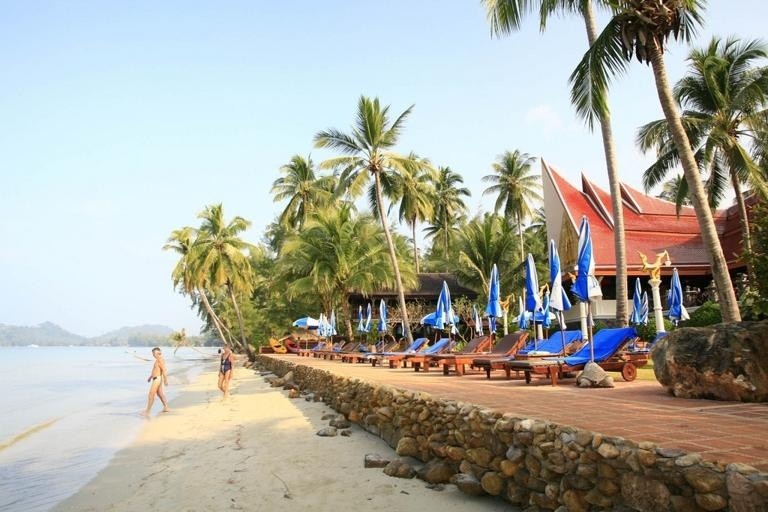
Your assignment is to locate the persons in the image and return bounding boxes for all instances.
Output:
[218,344,234,402]
[141,347,169,416]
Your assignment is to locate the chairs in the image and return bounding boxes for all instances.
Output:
[297,326,652,386]
